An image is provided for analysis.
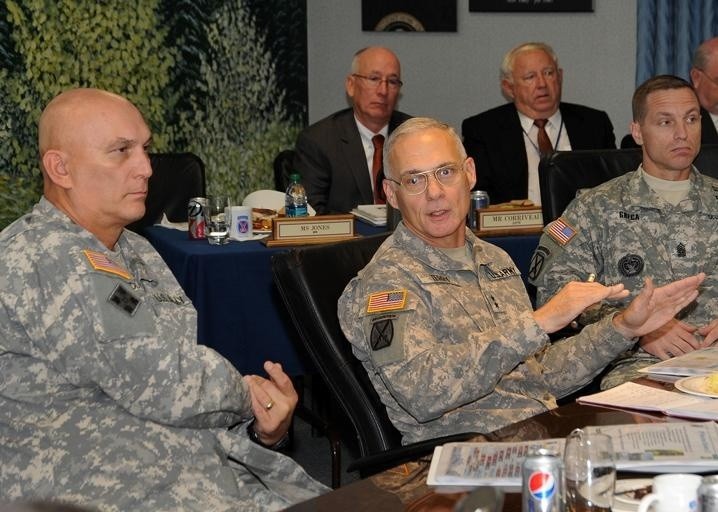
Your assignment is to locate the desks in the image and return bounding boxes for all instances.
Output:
[275,351,717,511]
[155,213,664,446]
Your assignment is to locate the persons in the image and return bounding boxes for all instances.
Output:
[619,34,718,207]
[533,77,711,388]
[617,42,718,171]
[462,43,618,245]
[294,46,436,237]
[0,87,331,512]
[340,116,709,445]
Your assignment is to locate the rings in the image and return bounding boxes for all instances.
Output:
[266,401,273,413]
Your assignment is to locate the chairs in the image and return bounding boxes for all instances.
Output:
[125,154,205,237]
[271,232,493,487]
[539,148,643,224]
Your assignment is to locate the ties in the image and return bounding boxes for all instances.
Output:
[372,135,386,205]
[533,119,554,159]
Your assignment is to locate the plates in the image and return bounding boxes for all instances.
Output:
[252,229,273,235]
[615,479,653,511]
[674,374,718,399]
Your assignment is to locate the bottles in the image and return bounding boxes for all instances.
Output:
[187,197,211,239]
[699,475,718,512]
[470,189,489,228]
[387,199,401,231]
[522,455,564,511]
[283,175,308,219]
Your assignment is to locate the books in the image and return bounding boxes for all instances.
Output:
[344,199,413,230]
[640,348,717,383]
[582,379,717,419]
[419,421,713,484]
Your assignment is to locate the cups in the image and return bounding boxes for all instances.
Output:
[563,433,616,512]
[639,474,704,512]
[224,206,253,241]
[205,196,233,244]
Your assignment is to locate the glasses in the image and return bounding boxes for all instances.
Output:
[351,73,404,90]
[699,69,717,83]
[386,163,464,196]
